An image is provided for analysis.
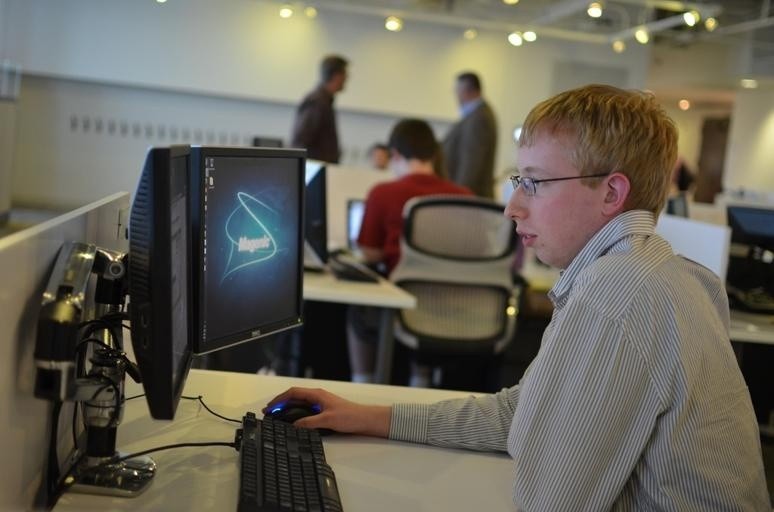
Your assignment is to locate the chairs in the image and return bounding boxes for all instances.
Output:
[386,192,530,389]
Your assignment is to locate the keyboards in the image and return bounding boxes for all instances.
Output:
[238,413,343,512]
[329,260,379,281]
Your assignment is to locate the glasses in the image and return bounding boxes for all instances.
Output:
[510,174,608,195]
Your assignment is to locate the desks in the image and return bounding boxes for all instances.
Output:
[45,367,518,512]
[301,248,417,384]
[727,306,773,437]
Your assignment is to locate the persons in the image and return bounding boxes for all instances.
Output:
[367,143,389,169]
[292,56,346,165]
[439,73,498,201]
[264,81,774,511]
[667,156,692,218]
[347,119,478,388]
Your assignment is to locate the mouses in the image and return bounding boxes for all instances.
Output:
[264,401,322,421]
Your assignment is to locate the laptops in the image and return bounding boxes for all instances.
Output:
[347,198,367,262]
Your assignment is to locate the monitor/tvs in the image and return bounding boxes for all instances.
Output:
[654,210,732,286]
[128,144,197,421]
[189,148,306,355]
